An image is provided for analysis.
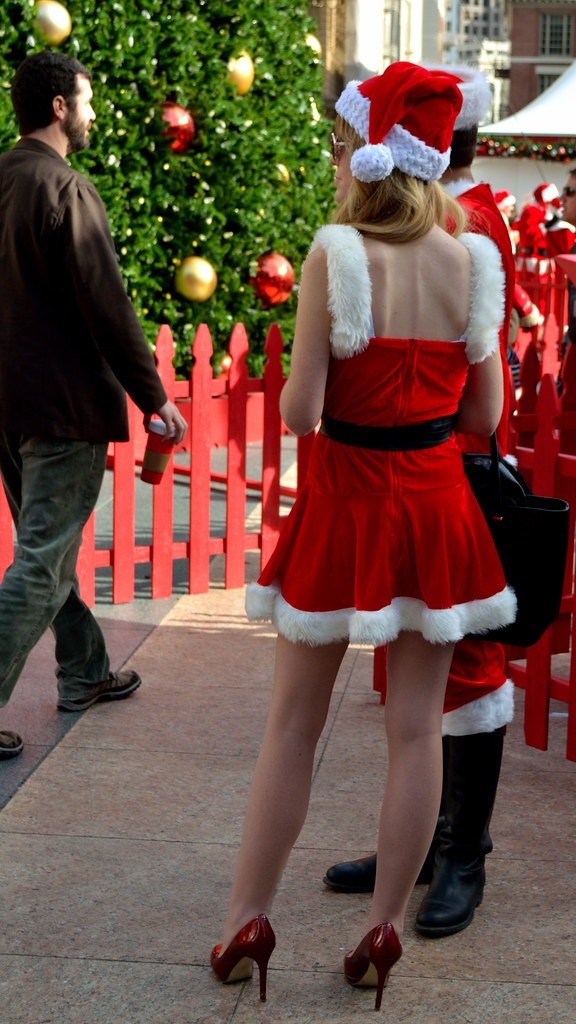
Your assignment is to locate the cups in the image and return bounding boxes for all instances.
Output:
[140,419,176,485]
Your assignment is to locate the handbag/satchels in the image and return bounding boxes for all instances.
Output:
[461,433,570,648]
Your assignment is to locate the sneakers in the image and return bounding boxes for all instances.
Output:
[57,670,141,711]
[0,730,24,755]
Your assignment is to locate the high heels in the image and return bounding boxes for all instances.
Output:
[343,922,403,1011]
[210,914,275,1001]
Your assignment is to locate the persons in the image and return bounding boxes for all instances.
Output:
[1,51,186,757]
[209,61,575,1012]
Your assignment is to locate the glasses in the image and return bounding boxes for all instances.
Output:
[563,187,576,197]
[330,133,347,160]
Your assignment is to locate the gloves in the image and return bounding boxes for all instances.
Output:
[520,304,544,331]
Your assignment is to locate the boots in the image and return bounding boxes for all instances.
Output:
[415,728,504,933]
[323,735,449,891]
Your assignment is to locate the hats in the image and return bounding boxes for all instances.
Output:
[421,64,494,131]
[335,62,464,184]
[534,183,559,203]
[494,191,516,210]
